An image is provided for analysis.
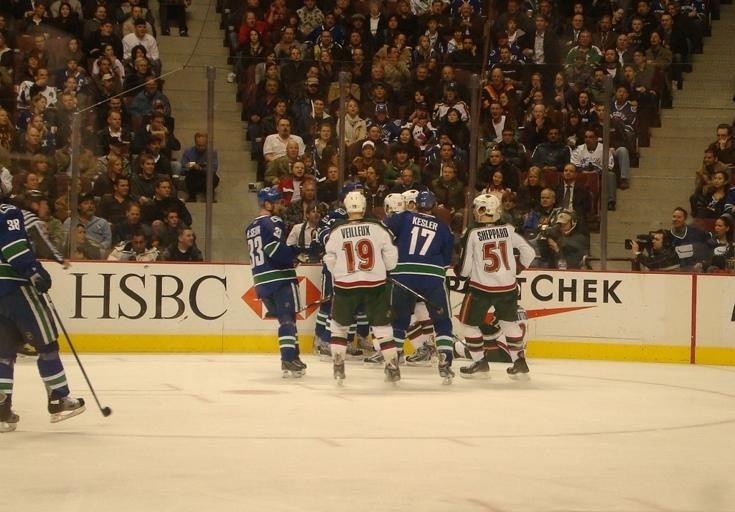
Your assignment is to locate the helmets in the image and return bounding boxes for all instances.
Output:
[259,187,281,208]
[344,190,437,217]
[25,191,42,202]
[474,194,501,223]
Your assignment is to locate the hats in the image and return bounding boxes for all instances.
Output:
[78,192,92,203]
[305,77,318,87]
[557,208,573,225]
[417,102,429,109]
[102,74,113,81]
[362,141,375,150]
[447,82,457,90]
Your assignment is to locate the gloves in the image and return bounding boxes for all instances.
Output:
[25,261,52,294]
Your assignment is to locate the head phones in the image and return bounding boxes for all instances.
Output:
[664,231,671,248]
[561,210,578,222]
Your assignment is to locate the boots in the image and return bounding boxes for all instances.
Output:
[334,352,344,380]
[45,384,85,413]
[385,359,400,381]
[507,351,529,374]
[438,352,455,377]
[460,357,489,374]
[0,392,19,423]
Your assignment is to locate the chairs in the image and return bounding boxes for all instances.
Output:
[514,91,735,271]
[215,1,269,192]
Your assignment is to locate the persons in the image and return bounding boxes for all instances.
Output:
[313,181,529,363]
[214,1,720,272]
[629,123,734,272]
[322,191,399,381]
[247,188,306,368]
[459,195,538,374]
[0,166,84,424]
[0,1,221,263]
[383,192,454,371]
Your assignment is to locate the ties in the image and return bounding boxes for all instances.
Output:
[564,185,571,208]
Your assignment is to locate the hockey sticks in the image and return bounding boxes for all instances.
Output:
[391,278,444,317]
[45,292,113,418]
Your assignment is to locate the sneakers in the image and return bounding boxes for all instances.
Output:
[620,180,629,189]
[281,358,306,372]
[607,202,615,210]
[320,336,437,363]
